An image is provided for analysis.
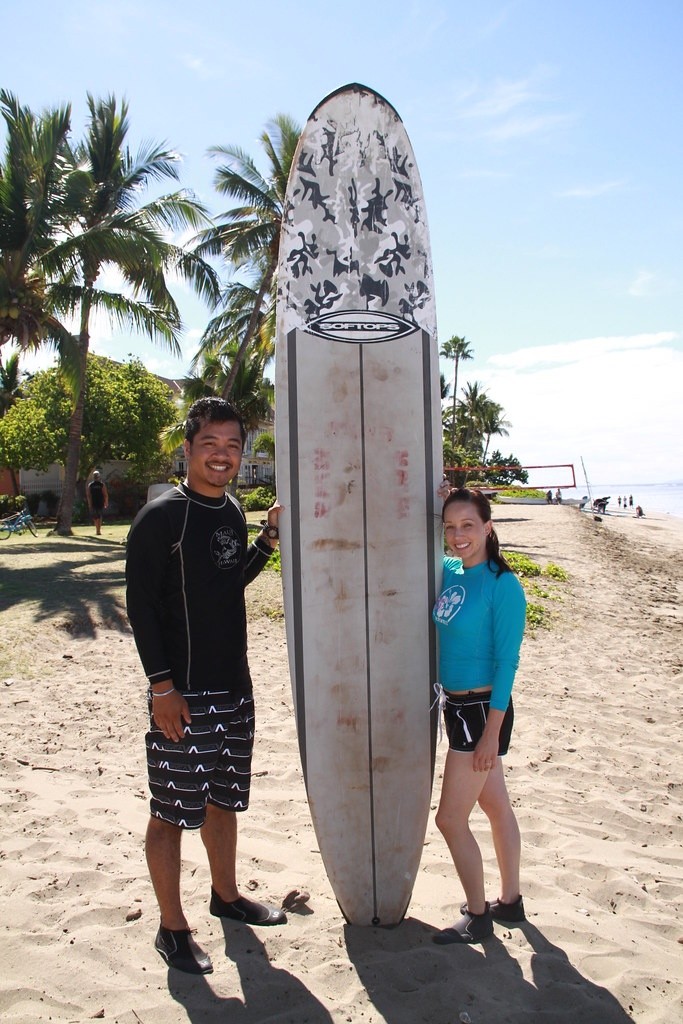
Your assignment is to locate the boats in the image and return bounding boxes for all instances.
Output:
[499,495,590,505]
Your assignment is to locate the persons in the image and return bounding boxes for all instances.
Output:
[593,496,610,514]
[636,505,646,518]
[429,473,526,945]
[125,396,289,976]
[618,494,633,509]
[546,488,562,505]
[86,471,108,535]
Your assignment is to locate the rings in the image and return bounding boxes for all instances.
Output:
[485,762,491,766]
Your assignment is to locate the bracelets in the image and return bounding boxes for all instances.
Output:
[152,687,176,696]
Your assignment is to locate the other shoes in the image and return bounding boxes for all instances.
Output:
[433,900,494,945]
[460,892,525,923]
[210,885,286,924]
[154,921,213,975]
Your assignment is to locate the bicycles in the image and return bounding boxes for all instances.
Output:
[0,504,39,540]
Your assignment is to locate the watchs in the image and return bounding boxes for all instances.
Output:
[262,522,279,539]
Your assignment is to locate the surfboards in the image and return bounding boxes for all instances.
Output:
[278,82,447,930]
